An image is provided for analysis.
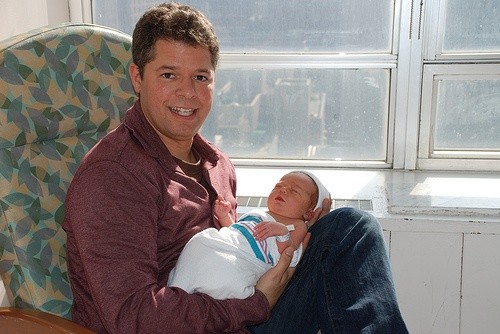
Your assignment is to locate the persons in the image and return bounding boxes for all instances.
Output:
[61,3,408,334]
[167,171,330,300]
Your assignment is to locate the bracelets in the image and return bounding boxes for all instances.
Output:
[277,232,290,242]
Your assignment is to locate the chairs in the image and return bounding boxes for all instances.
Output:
[0,22,133,334]
